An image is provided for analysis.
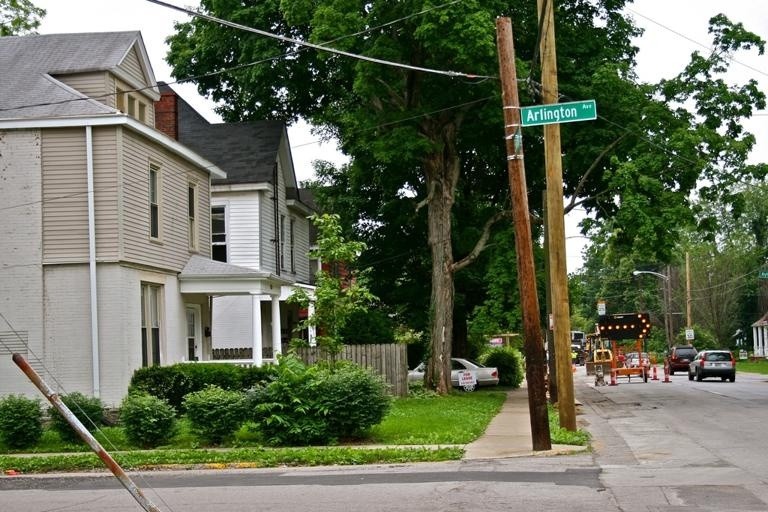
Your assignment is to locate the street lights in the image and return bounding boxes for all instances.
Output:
[633,270,674,345]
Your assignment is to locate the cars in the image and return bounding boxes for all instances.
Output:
[408,357,498,393]
[664,343,737,381]
